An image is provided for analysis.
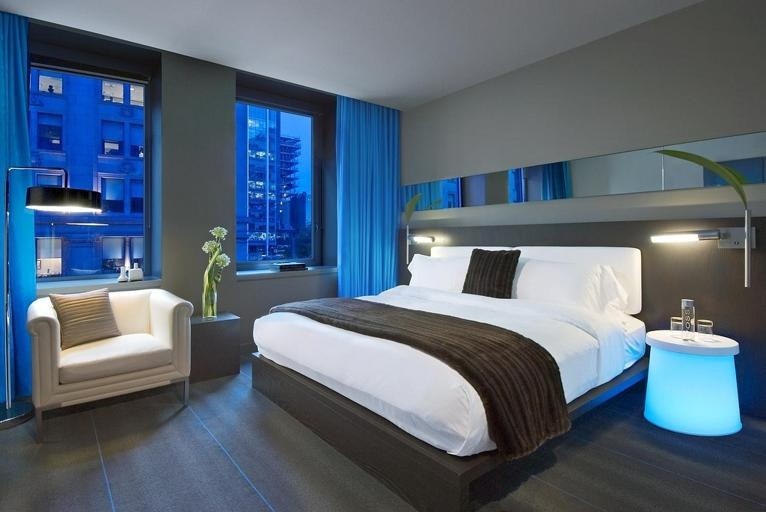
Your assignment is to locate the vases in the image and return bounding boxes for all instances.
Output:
[202,292,218,321]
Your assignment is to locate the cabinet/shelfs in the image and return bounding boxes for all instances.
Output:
[191,312,240,383]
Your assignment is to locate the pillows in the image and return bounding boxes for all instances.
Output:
[48,288,121,350]
[461,248,521,299]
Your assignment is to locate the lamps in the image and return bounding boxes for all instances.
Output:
[649,230,728,244]
[0,167,102,431]
[408,235,435,244]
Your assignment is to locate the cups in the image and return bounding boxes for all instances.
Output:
[670,316,682,338]
[697,319,713,342]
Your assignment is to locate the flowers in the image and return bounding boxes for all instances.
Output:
[202,225,231,318]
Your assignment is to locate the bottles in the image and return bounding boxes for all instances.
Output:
[681,299,696,340]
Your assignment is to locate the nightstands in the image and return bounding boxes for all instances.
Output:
[643,330,742,436]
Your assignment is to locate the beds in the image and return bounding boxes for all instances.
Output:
[252,246,649,512]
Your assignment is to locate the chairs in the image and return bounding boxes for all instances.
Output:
[26,289,194,445]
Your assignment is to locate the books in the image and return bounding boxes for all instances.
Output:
[269,261,308,272]
